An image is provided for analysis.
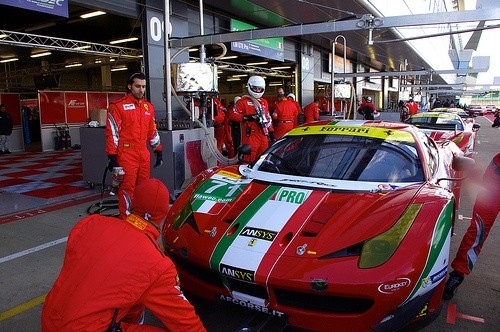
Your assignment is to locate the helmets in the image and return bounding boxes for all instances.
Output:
[287,93,295,101]
[366,96,371,102]
[247,76,265,98]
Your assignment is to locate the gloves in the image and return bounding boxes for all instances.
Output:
[244,113,259,121]
[153,151,162,168]
[106,156,119,172]
[269,131,274,143]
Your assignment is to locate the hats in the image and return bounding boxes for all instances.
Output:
[133,178,170,219]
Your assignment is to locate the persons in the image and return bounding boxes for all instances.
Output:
[40,178,208,332]
[442,152,500,302]
[195,76,500,166]
[0,103,13,155]
[104,72,163,220]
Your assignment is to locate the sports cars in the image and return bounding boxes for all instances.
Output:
[161,119,467,332]
[402,106,479,159]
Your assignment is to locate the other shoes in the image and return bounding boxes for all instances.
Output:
[443,272,465,297]
[0,150,3,154]
[4,150,11,154]
